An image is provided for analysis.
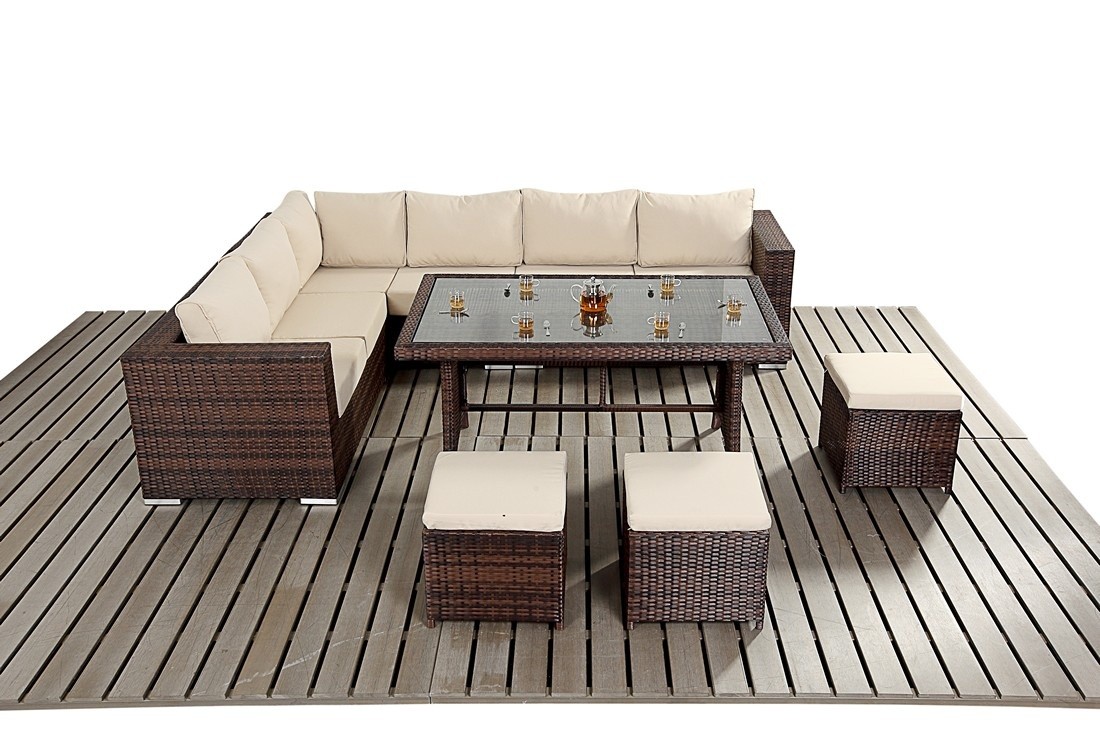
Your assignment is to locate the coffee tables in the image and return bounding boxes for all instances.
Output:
[394,274,794,453]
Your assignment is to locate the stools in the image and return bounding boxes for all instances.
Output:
[423,452,568,632]
[821,354,965,497]
[622,451,773,634]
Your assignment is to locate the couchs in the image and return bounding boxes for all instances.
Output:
[123,187,797,504]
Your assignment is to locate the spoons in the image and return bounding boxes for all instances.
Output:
[647,284,653,291]
[717,300,747,306]
[439,308,469,313]
[544,320,551,335]
[678,322,686,337]
[504,284,511,291]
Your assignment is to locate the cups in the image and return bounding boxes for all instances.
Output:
[450,290,464,309]
[727,293,746,312]
[726,312,742,328]
[647,312,671,330]
[519,275,540,291]
[660,290,681,306]
[661,274,681,291]
[513,329,535,341]
[520,291,539,305]
[647,329,669,342]
[451,308,464,323]
[511,312,534,330]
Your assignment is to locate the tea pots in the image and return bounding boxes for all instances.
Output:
[571,276,616,313]
[571,309,618,339]
